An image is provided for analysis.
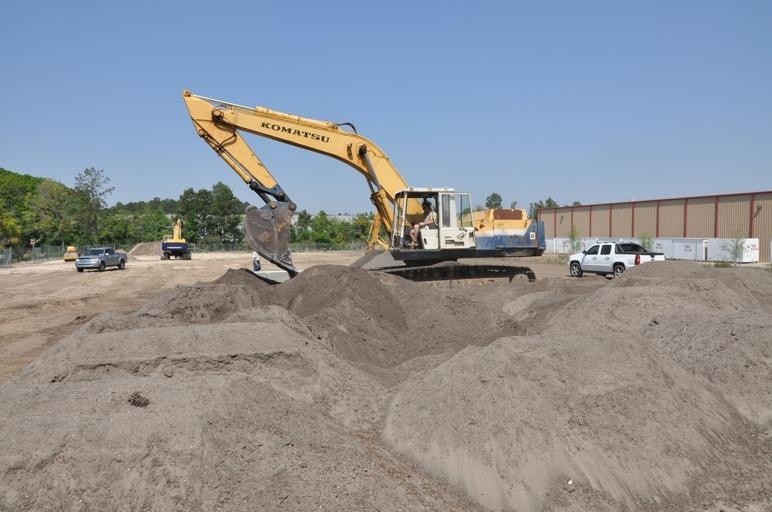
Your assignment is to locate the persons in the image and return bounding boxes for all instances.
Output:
[408,200,438,249]
[253,251,261,271]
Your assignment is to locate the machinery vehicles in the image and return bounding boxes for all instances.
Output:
[64,246,78,261]
[162,217,191,260]
[182,89,547,287]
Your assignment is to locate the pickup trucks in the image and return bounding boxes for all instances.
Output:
[568,242,665,278]
[76,247,127,272]
